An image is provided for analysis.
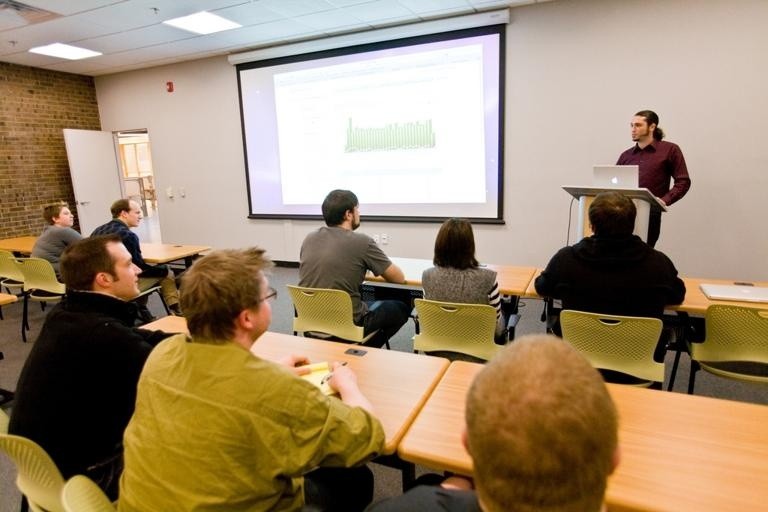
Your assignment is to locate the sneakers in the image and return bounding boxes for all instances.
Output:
[138,309,156,322]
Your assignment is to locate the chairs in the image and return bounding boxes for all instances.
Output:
[285,283,391,349]
[0,248,32,321]
[21,257,67,342]
[410,297,505,361]
[687,305,768,394]
[0,409,65,512]
[558,308,666,391]
[61,474,119,512]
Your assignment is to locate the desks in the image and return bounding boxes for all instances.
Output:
[397,361,767,512]
[525,267,768,392]
[137,315,451,494]
[0,236,212,270]
[363,257,537,340]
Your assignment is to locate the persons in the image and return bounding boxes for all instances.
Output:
[365,333,619,511]
[9,236,184,503]
[111,250,383,512]
[616,109,692,249]
[88,199,183,315]
[532,191,686,388]
[421,219,508,343]
[26,202,85,285]
[294,189,416,349]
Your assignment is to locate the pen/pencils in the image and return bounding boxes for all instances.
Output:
[321,361,348,385]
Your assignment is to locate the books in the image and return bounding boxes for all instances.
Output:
[287,361,336,396]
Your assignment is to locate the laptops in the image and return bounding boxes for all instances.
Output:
[594,165,640,189]
